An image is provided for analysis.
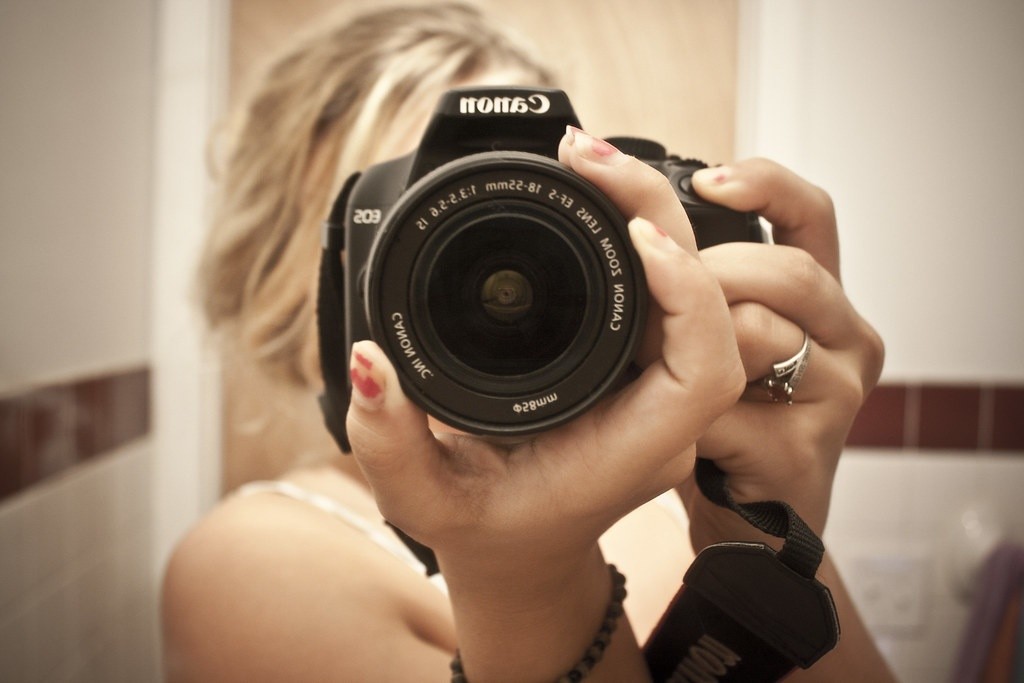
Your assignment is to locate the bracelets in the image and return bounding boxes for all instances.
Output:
[448,562,627,683]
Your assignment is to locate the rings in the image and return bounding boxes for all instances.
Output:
[759,330,811,406]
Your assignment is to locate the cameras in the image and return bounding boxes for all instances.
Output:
[346,87,766,441]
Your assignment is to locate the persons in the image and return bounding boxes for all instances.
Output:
[159,0,900,683]
